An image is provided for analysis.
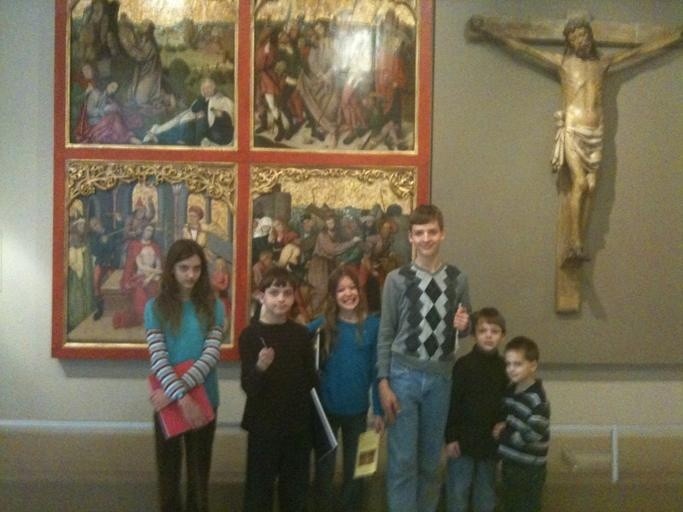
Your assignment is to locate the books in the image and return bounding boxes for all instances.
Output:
[306,385,339,463]
[146,357,216,441]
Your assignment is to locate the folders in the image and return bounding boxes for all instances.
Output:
[309,387,337,464]
[146,359,215,438]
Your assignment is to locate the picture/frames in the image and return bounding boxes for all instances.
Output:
[49,0,434,360]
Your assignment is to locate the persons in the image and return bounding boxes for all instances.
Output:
[466,15,683,272]
[373,204,475,511]
[236,264,317,512]
[440,307,513,512]
[302,264,386,511]
[489,337,552,510]
[141,238,225,511]
